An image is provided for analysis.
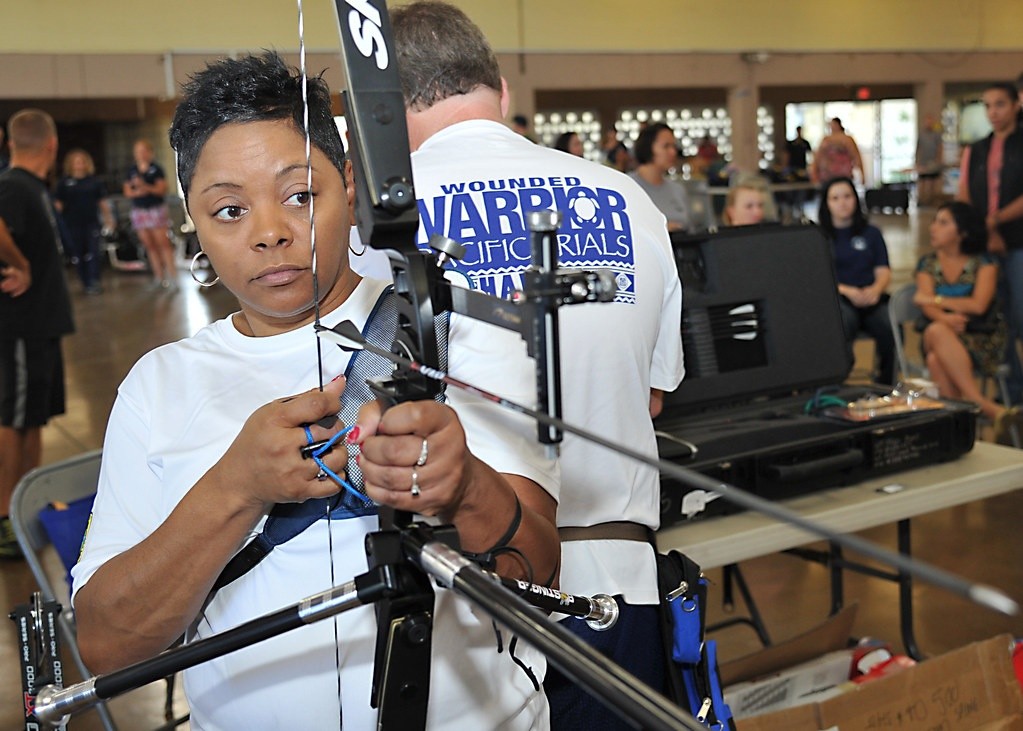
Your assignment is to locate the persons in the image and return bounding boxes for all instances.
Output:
[1014,71,1023,115]
[0,111,77,560]
[343,1,700,731]
[555,131,586,159]
[784,125,812,183]
[512,114,538,143]
[121,140,179,297]
[697,133,719,159]
[599,124,630,172]
[58,151,113,296]
[71,52,562,731]
[816,176,905,390]
[911,200,1013,441]
[707,173,815,235]
[813,116,864,189]
[635,123,695,231]
[955,81,1023,399]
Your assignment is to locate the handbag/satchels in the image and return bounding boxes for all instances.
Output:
[551,521,739,731]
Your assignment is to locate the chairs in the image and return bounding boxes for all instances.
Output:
[8,446,195,731]
[888,279,1023,451]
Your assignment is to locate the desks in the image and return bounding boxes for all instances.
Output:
[704,181,824,224]
[654,438,1023,664]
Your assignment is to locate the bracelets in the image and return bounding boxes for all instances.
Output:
[936,295,943,305]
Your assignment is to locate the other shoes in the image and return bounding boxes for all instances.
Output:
[0,518,19,556]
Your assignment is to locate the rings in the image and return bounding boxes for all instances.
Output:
[412,467,420,497]
[417,437,428,466]
[317,455,327,481]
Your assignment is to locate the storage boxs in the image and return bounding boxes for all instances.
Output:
[716,602,1023,731]
